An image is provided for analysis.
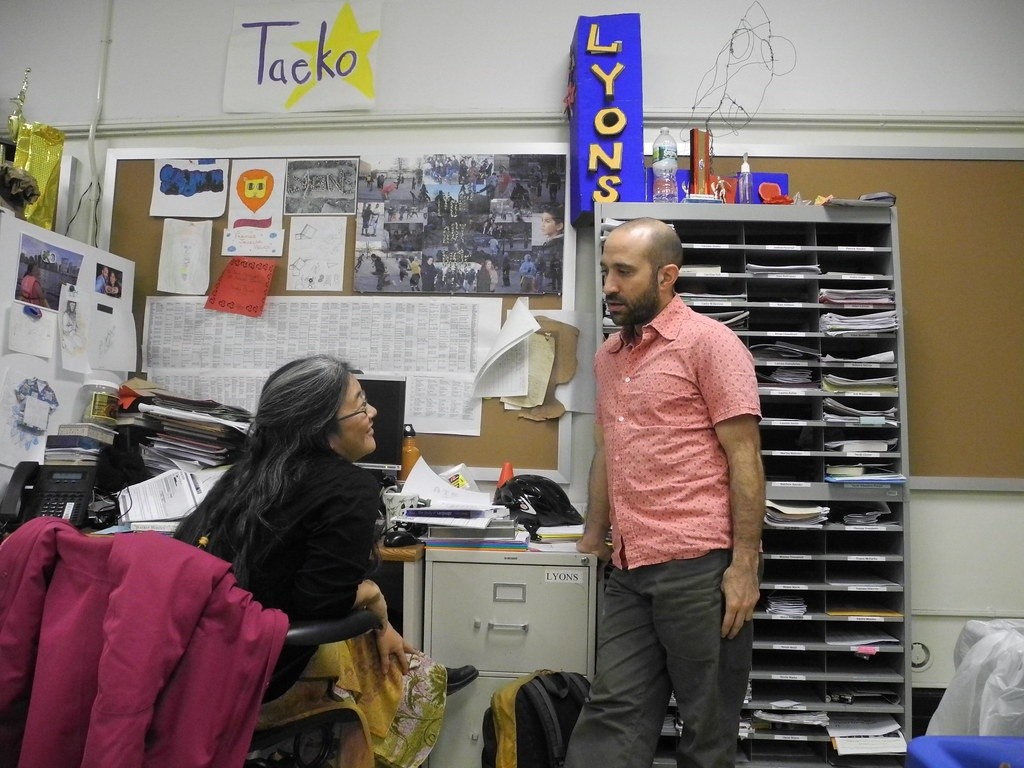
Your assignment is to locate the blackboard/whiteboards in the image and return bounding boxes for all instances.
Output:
[2,3,1024,139]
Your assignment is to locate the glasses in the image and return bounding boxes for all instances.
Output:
[336,391,367,422]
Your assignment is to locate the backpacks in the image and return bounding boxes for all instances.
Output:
[481,668,591,768]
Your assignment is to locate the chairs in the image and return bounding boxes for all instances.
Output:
[0,517,376,768]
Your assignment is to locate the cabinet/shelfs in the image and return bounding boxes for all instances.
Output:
[423,542,598,768]
[594,201,911,760]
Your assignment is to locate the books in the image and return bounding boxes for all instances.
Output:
[699,311,749,328]
[131,522,180,532]
[680,265,721,273]
[825,438,898,452]
[44,448,99,465]
[537,526,614,543]
[826,463,863,475]
[116,393,256,475]
[405,509,529,551]
[767,596,806,616]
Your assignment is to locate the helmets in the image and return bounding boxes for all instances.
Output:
[492,474,584,526]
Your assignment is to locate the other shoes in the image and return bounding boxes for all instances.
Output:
[446,665,479,696]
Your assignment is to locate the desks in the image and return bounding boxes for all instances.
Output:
[79,525,424,650]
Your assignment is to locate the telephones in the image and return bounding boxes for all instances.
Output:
[0,460,100,530]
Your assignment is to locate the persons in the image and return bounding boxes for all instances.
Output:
[355,155,563,291]
[170,354,480,768]
[715,176,731,203]
[96,267,121,298]
[565,218,766,768]
[22,263,50,308]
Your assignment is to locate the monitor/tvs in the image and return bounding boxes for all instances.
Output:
[351,374,406,481]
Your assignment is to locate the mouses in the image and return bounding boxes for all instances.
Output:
[383,531,418,548]
